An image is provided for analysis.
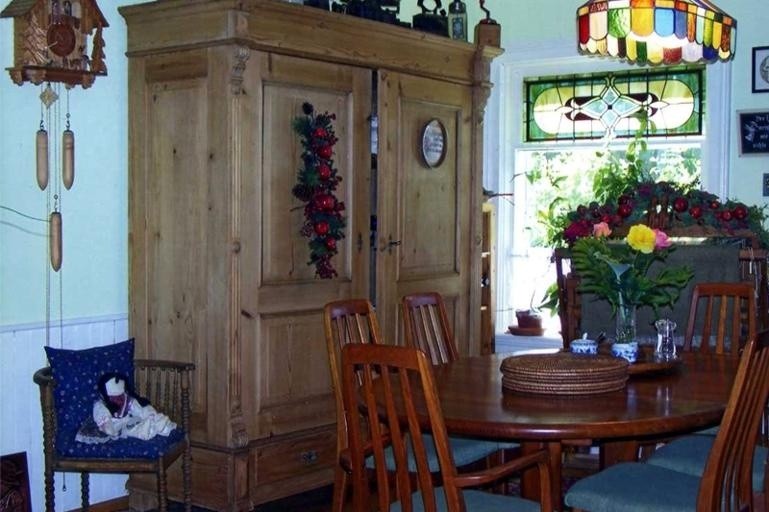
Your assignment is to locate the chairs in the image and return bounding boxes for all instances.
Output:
[34,360,196,512]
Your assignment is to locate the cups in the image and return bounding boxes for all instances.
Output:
[612,342,639,361]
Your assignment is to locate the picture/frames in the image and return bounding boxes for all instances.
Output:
[736,110,769,157]
[752,47,769,93]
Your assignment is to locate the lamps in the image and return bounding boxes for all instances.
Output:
[576,0,737,66]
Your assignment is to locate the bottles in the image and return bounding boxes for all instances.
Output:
[447,2,467,43]
[570,332,599,353]
[653,319,679,358]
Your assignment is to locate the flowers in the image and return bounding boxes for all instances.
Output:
[563,223,695,342]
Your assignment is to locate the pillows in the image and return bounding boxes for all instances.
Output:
[44,337,135,457]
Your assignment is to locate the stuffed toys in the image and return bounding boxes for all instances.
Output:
[91,370,177,440]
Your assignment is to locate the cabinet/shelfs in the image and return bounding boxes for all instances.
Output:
[117,0,505,512]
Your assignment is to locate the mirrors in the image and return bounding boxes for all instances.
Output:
[420,117,447,169]
